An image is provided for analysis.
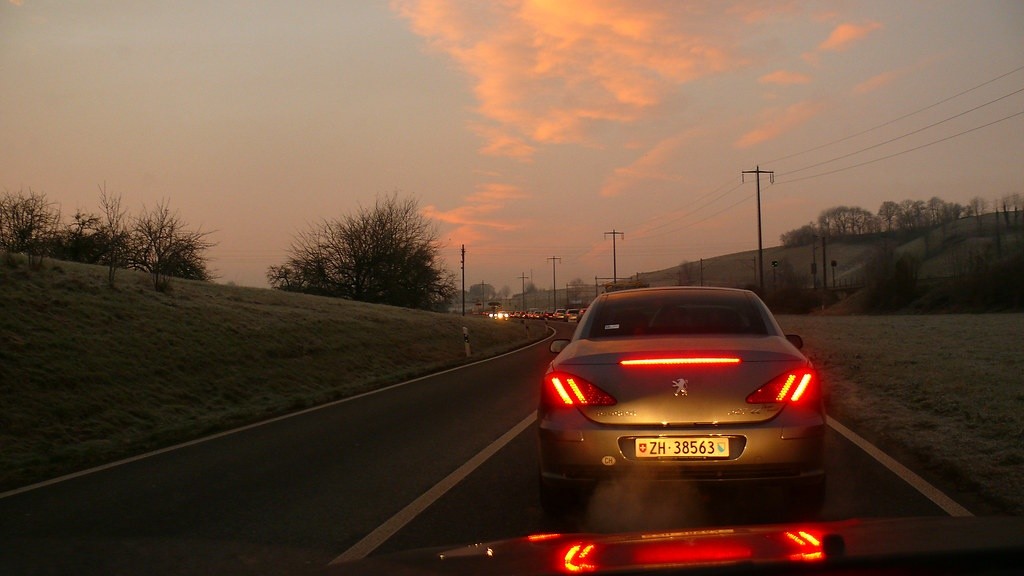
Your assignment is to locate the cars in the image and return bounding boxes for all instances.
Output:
[552,309,566,320]
[564,309,579,322]
[480,311,554,320]
[539,286,827,482]
[577,308,587,323]
